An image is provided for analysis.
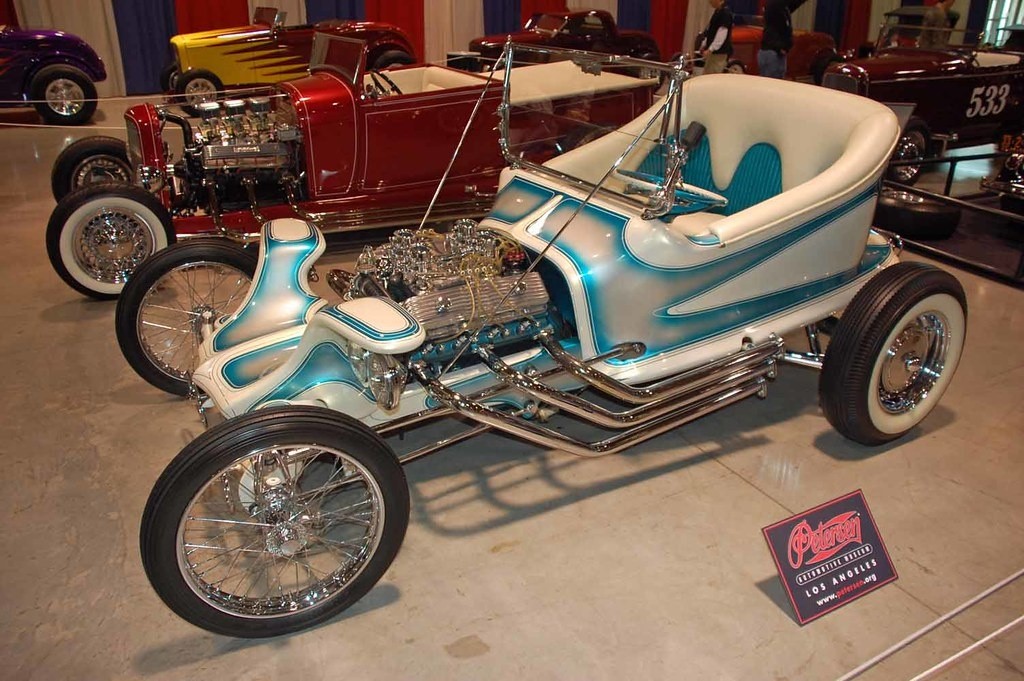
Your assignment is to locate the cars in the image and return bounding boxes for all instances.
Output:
[115,33,969,642]
[0,1,1024,301]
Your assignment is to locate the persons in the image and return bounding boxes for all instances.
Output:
[697,0,735,75]
[758,0,805,79]
